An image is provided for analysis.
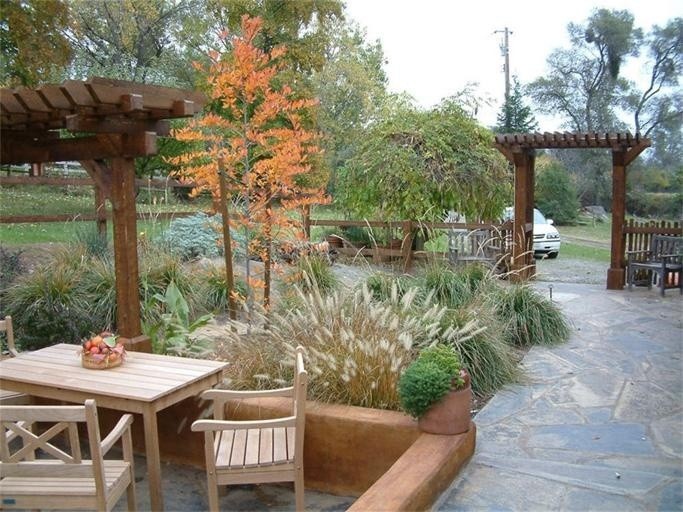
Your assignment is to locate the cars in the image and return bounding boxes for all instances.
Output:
[499,206,560,258]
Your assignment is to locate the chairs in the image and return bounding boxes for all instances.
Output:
[190,346,308,512]
[0,399,136,512]
[624,233,683,298]
[0,315,38,449]
[448,229,496,277]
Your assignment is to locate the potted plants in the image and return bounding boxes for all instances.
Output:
[398,342,470,435]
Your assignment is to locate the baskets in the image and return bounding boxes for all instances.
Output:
[80,347,125,369]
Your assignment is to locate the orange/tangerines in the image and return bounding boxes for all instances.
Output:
[91,335,102,346]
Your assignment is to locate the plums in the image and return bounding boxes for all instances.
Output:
[99,344,108,351]
[90,346,100,353]
[101,348,109,353]
[83,341,91,350]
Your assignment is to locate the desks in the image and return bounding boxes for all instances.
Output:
[0,341,231,511]
